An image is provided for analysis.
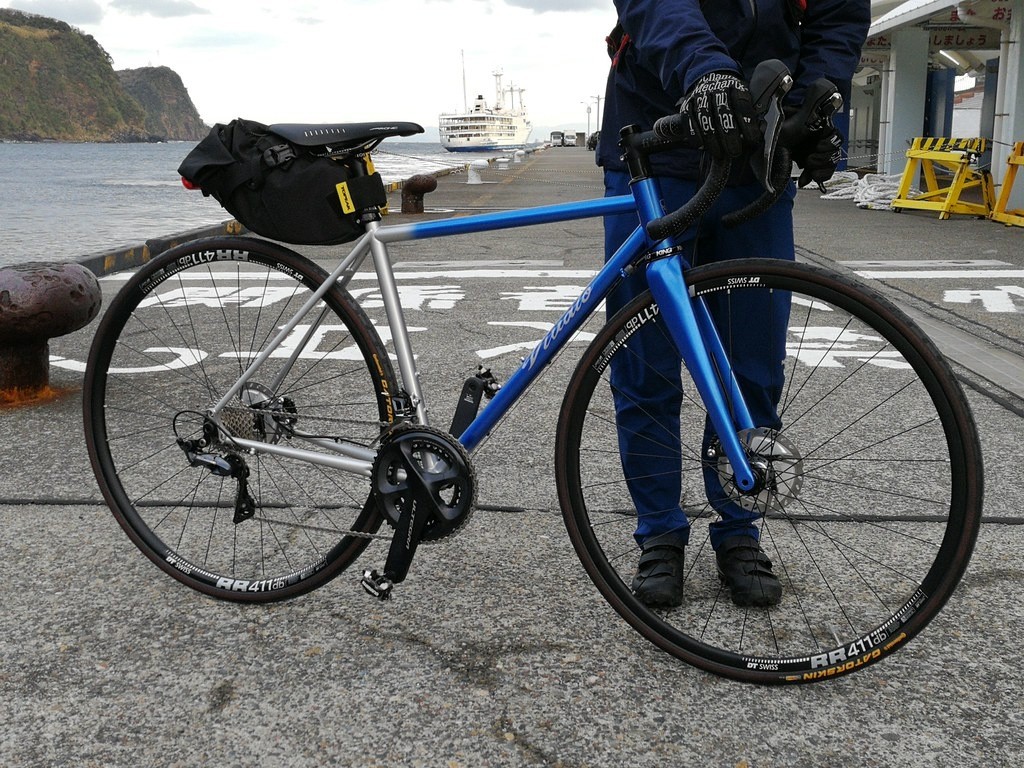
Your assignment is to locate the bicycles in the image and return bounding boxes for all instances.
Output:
[81,59,986,688]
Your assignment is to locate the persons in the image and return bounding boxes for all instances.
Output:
[596,0,875,608]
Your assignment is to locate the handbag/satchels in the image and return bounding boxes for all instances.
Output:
[177,118,387,245]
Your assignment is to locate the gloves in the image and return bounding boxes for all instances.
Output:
[680,74,764,158]
[796,128,844,189]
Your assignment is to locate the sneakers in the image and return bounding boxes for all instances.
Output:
[631,536,684,607]
[715,535,783,606]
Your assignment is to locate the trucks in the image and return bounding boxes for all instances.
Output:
[550,131,563,147]
[563,128,578,147]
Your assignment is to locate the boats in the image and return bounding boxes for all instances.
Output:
[439,49,532,152]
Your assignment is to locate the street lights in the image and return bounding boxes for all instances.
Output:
[590,94,600,132]
[581,102,591,138]
